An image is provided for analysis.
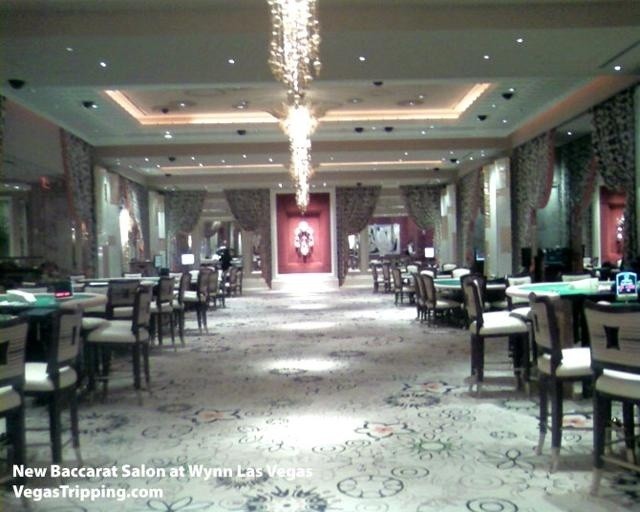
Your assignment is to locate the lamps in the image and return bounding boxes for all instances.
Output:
[268,96,343,216]
[267,1,322,108]
